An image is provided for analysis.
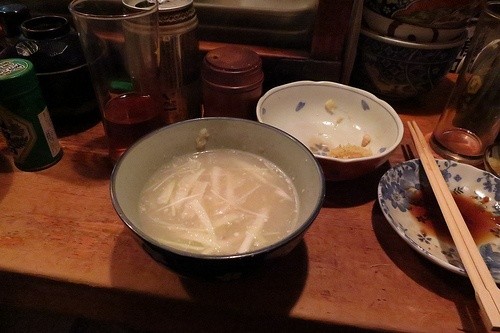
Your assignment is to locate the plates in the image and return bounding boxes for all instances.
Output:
[378,158,500,285]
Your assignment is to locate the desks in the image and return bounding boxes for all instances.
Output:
[0,33,500,333]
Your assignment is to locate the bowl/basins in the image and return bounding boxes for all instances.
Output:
[256,81,404,182]
[359,0,482,97]
[109,116,325,282]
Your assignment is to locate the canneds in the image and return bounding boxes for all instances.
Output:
[121,0,199,126]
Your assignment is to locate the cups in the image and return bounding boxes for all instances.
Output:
[429,8,500,167]
[69,0,165,170]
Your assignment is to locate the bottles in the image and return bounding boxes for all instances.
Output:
[0,59,64,172]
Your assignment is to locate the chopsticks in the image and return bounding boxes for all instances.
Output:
[406,120,500,333]
